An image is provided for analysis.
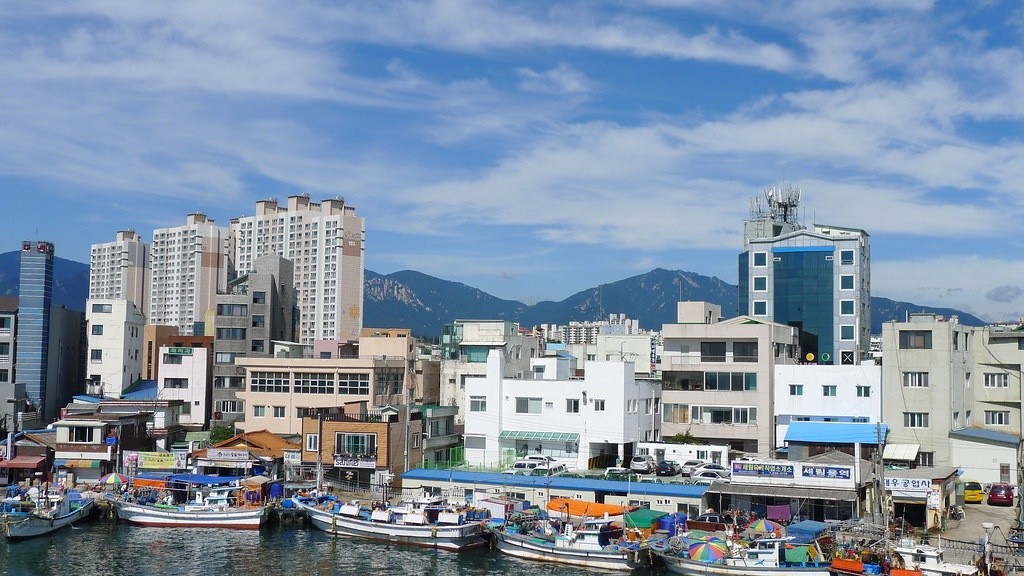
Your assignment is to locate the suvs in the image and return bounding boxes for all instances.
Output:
[629,454,656,474]
[500,453,569,479]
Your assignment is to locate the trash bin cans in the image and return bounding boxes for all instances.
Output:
[659,512,687,537]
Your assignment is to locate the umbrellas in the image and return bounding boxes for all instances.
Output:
[689,536,727,576]
[99,471,131,494]
[750,517,784,539]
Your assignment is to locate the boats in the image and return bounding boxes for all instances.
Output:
[647,519,1013,576]
[0,486,94,543]
[496,498,650,572]
[291,488,491,554]
[102,471,276,531]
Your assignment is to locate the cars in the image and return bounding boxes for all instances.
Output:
[688,463,731,477]
[987,483,1014,507]
[963,481,985,504]
[603,467,713,487]
[656,460,682,477]
[690,470,731,486]
[681,459,705,477]
